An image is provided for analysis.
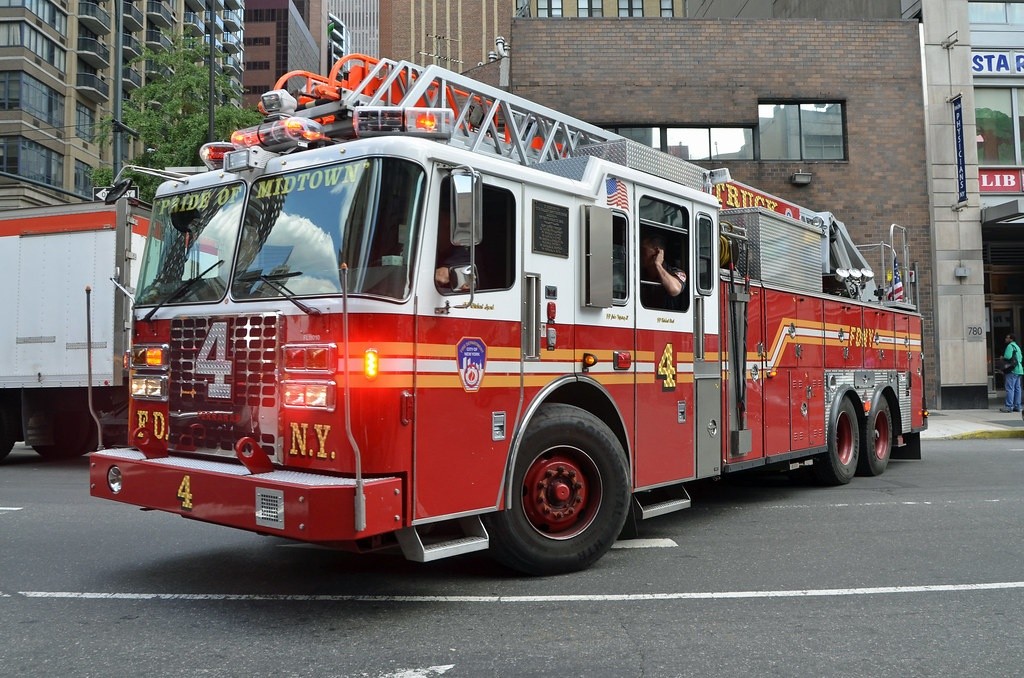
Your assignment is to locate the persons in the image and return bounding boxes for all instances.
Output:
[999,333,1023,413]
[640,232,687,310]
[433,208,476,292]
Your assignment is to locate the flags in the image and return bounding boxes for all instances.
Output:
[886,257,904,302]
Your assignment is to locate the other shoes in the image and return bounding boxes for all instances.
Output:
[1000,408,1013,413]
[1013,408,1023,412]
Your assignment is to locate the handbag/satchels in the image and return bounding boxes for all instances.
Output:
[1000,344,1018,374]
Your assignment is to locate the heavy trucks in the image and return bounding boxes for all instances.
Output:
[0,197,220,460]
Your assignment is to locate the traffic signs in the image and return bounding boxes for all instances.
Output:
[93,186,139,202]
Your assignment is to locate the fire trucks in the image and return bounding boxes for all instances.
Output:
[82,53,929,578]
